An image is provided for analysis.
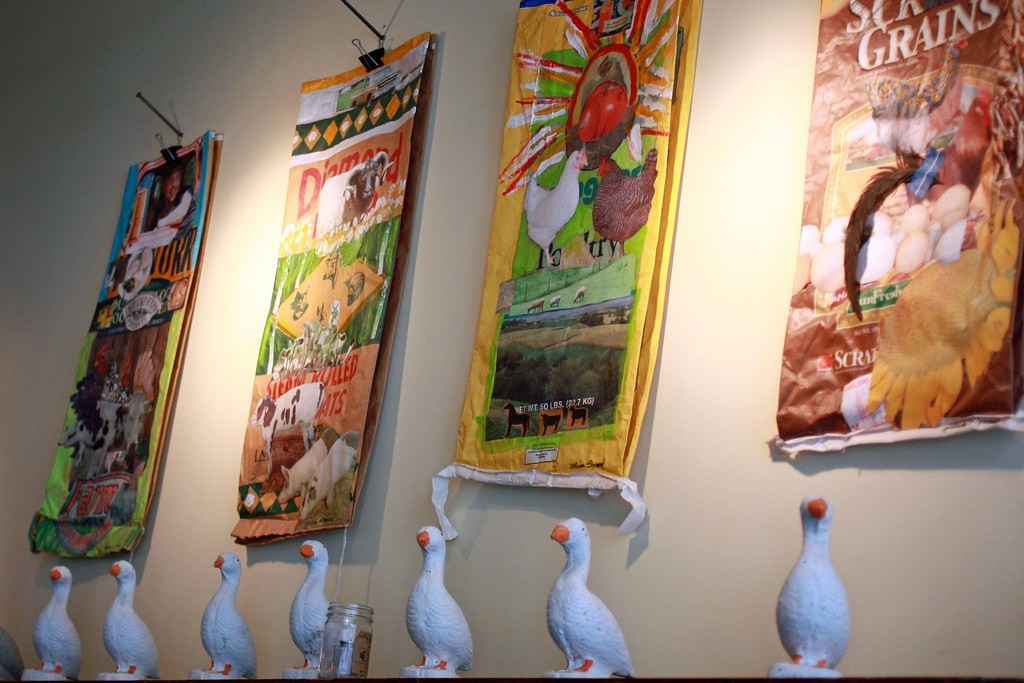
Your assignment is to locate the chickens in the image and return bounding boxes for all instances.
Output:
[524,148,589,268]
[593,147,658,255]
[863,34,971,157]
[844,86,995,321]
[95,345,154,402]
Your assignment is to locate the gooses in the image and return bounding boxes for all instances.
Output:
[776,495,852,669]
[289,539,335,669]
[32,565,83,681]
[405,524,474,673]
[546,516,636,677]
[199,551,259,679]
[101,560,162,679]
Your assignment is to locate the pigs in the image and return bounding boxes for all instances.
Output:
[58,392,155,474]
[297,430,361,520]
[278,426,340,513]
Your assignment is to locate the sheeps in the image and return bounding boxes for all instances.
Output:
[573,286,586,303]
[528,298,544,313]
[569,405,587,427]
[550,295,561,307]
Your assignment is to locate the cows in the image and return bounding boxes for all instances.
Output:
[541,413,561,436]
[250,382,325,477]
[313,151,394,243]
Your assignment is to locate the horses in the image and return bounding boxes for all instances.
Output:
[503,401,530,438]
